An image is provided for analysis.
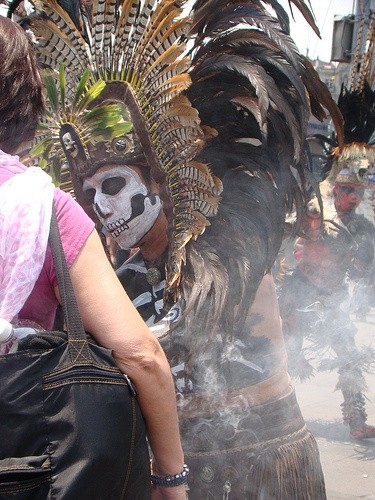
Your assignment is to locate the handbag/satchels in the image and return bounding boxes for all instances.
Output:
[0,196,152,500]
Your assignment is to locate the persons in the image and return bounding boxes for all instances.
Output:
[279,167,374,441]
[0,15,188,500]
[57,97,327,500]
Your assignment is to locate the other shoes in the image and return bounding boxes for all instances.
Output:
[350,423,375,439]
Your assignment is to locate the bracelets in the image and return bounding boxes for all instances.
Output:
[151,464,190,488]
